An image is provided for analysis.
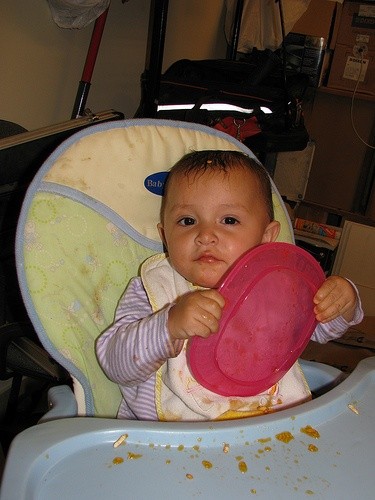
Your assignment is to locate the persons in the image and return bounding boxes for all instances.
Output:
[93,150,365,423]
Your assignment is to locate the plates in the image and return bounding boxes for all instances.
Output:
[186,241,326,396]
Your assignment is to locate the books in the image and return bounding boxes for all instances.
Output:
[292,218,342,249]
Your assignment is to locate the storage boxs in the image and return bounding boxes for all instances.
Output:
[327,44,375,98]
[336,1,375,50]
